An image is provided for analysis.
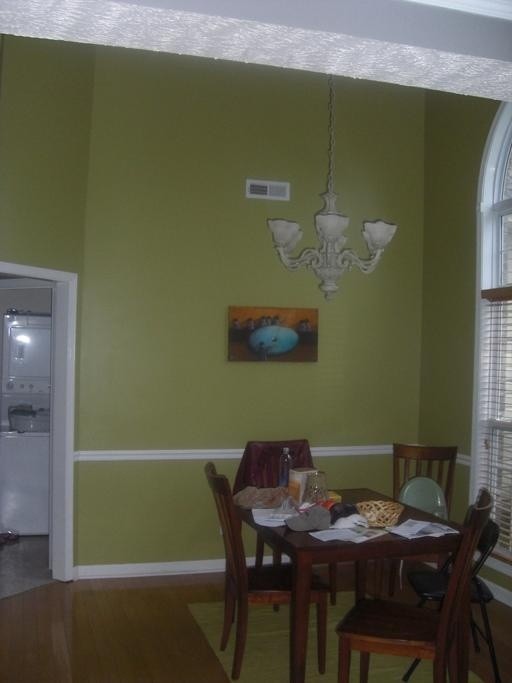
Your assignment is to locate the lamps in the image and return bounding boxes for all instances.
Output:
[265,74,398,302]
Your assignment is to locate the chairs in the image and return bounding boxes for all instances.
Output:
[401,516,501,683]
[203,459,330,682]
[244,437,338,611]
[373,438,459,600]
[332,485,495,683]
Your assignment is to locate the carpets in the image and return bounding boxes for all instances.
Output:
[0,533,56,601]
[188,587,488,683]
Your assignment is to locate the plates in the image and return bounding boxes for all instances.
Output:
[396,475,446,519]
[249,324,301,356]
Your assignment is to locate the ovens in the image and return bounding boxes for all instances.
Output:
[1,313,49,539]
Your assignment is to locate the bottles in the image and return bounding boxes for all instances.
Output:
[232,315,316,333]
[278,446,293,488]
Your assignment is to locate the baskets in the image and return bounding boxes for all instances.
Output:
[354,499,405,527]
